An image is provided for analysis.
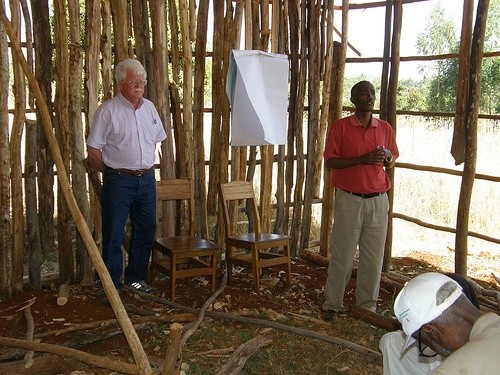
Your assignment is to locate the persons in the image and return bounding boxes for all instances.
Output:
[86,59,167,306]
[379,272,500,375]
[323,81,400,323]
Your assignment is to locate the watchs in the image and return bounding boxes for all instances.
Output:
[386,155,393,163]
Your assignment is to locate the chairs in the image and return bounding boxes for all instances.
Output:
[219,180,292,296]
[155,178,221,304]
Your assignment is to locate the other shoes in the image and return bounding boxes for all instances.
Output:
[123,280,156,295]
[324,310,335,321]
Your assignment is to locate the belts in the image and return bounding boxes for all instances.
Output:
[344,190,382,199]
[126,170,146,176]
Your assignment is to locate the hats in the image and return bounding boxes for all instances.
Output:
[393,272,463,360]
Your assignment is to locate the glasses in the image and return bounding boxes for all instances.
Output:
[419,322,438,358]
[121,79,148,86]
